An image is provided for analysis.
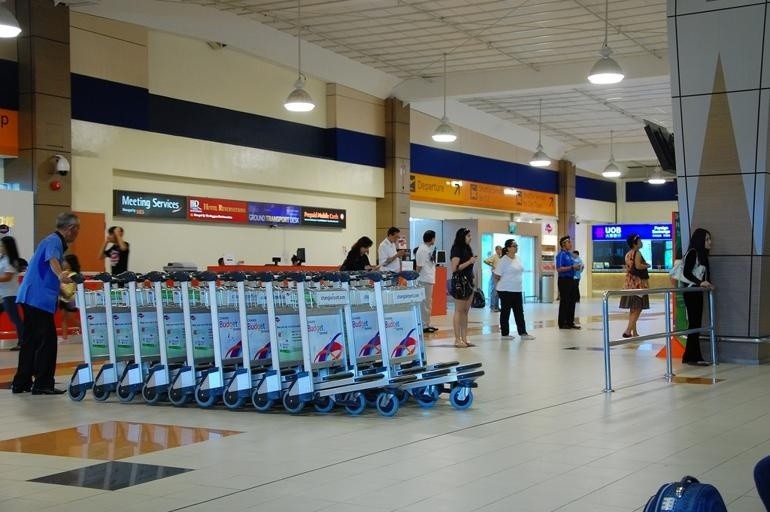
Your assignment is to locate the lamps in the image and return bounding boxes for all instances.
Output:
[602,131,621,178]
[0,6,22,39]
[587,0,625,86]
[529,100,551,167]
[647,158,667,184]
[283,0,316,111]
[432,54,457,143]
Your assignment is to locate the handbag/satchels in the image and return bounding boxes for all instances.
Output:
[471,286,485,309]
[628,250,651,280]
[669,248,708,285]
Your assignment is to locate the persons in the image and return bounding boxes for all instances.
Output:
[493,238,537,340]
[483,245,503,312]
[570,251,584,303]
[337,235,380,270]
[619,233,651,338]
[415,230,439,334]
[377,228,406,274]
[17,256,29,272]
[0,236,25,351]
[556,236,580,330]
[682,228,716,366]
[94,225,130,275]
[11,212,79,395]
[59,254,85,344]
[451,227,480,348]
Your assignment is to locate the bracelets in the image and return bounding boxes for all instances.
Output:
[572,265,575,270]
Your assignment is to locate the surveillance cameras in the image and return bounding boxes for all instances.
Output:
[57,160,70,176]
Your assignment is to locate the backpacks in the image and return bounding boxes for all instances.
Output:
[449,265,473,300]
[641,475,728,512]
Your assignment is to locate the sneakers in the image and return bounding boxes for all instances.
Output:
[464,342,476,347]
[521,334,537,341]
[455,340,468,348]
[501,335,515,340]
[9,346,22,352]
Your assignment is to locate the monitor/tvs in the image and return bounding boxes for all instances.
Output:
[435,250,446,265]
[296,248,305,263]
[642,121,674,173]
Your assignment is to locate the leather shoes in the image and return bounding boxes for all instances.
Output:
[622,333,633,339]
[12,383,31,393]
[428,326,439,331]
[32,385,67,395]
[681,358,713,366]
[559,321,581,329]
[423,328,434,333]
